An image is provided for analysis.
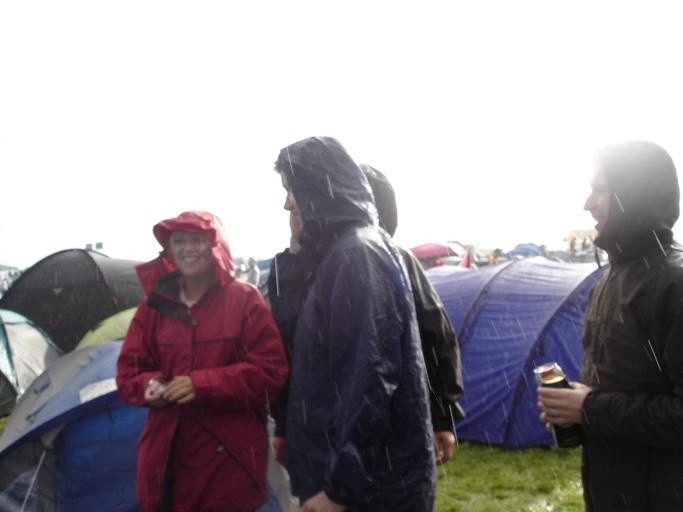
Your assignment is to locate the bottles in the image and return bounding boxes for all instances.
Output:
[534,361,586,448]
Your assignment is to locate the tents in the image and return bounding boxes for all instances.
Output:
[0,341,284,512]
[0,244,158,413]
[408,240,483,268]
[416,256,611,450]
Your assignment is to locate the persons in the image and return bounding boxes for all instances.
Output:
[462,245,475,267]
[564,234,593,258]
[533,138,683,512]
[487,248,501,265]
[113,209,286,512]
[227,255,261,286]
